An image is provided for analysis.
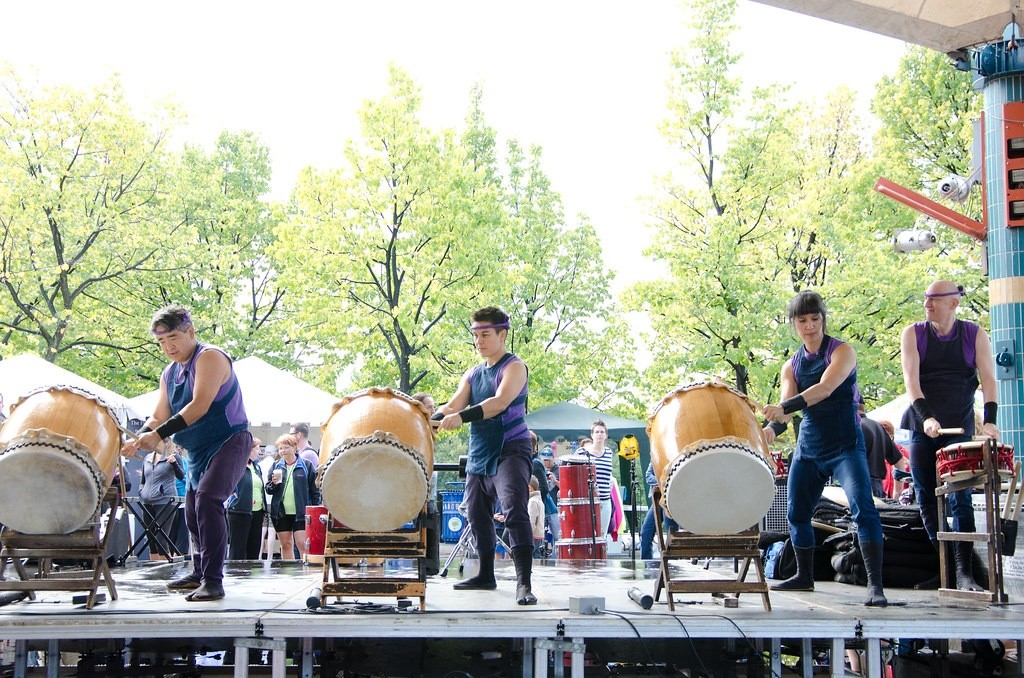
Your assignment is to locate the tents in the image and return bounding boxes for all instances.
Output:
[866,386,984,441]
[0,352,127,422]
[118,354,345,452]
[526,400,652,534]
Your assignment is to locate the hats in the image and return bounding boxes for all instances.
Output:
[539,446,554,462]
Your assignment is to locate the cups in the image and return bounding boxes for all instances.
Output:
[273,470,282,483]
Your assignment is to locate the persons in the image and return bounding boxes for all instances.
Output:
[637,463,679,561]
[758,288,889,605]
[428,305,536,605]
[120,302,255,601]
[899,279,999,588]
[227,422,320,563]
[878,419,912,498]
[528,419,613,560]
[856,394,904,501]
[491,500,511,559]
[103,429,191,559]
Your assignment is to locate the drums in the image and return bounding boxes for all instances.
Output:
[0,383,124,534]
[934,440,1016,482]
[646,380,778,535]
[555,454,608,559]
[302,503,384,566]
[315,387,436,534]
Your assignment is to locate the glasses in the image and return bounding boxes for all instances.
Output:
[540,451,554,456]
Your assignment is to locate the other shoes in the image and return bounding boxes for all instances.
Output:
[168,572,202,589]
[187,583,225,602]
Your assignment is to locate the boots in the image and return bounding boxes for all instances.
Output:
[955,541,984,591]
[454,549,497,590]
[914,538,954,590]
[859,541,886,606]
[512,544,538,605]
[771,543,814,591]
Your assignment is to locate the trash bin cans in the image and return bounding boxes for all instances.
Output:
[437,481,466,544]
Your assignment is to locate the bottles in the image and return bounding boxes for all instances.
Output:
[359,558,367,575]
